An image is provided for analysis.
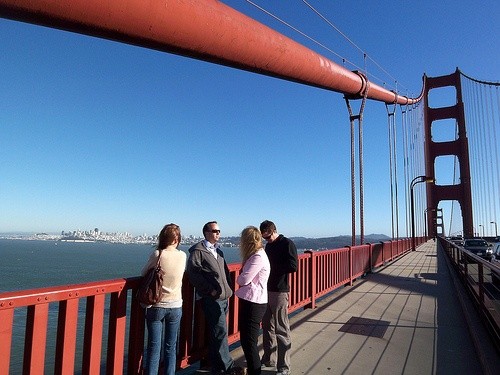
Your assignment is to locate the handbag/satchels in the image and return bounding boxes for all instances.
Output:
[135,249,165,305]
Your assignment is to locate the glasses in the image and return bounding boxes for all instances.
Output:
[262,234,272,239]
[207,230,220,233]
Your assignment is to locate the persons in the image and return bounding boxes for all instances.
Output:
[186,221,245,375]
[259,220,299,375]
[235,225,271,375]
[141,223,187,375]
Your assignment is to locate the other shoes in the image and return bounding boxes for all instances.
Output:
[260,360,276,367]
[276,369,290,375]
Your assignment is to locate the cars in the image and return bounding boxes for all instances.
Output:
[449,234,500,289]
